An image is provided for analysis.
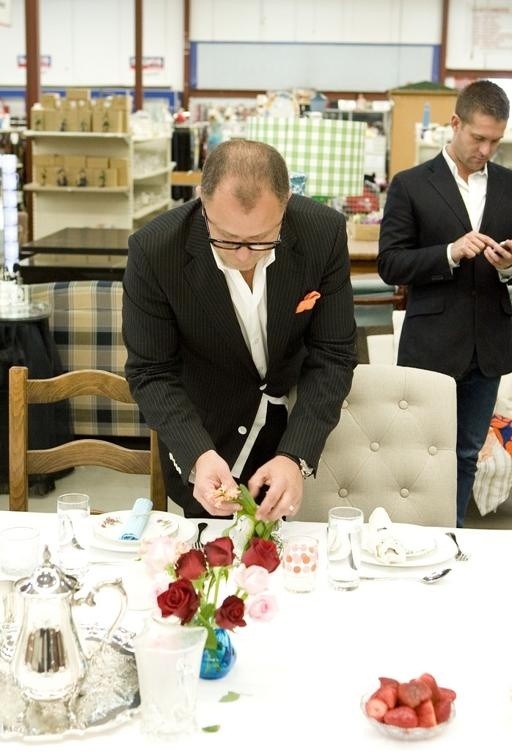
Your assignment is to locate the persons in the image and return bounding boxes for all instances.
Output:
[122,139,358,522]
[376,80,512,527]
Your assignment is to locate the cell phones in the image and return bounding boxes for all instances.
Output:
[492,239,509,255]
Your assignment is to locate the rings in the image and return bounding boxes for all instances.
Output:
[288,504,296,515]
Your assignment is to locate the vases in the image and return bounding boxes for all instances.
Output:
[199,622,240,682]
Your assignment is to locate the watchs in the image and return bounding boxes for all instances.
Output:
[277,453,315,479]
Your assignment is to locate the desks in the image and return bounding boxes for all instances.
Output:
[9,219,169,446]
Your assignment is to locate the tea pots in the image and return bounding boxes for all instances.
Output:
[1,545,128,706]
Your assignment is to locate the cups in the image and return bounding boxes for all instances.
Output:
[327,504,361,591]
[284,536,319,591]
[135,628,216,713]
[56,493,90,542]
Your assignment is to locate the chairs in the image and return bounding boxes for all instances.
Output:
[277,358,463,532]
[1,361,176,515]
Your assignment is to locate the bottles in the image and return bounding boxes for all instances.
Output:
[29,93,127,135]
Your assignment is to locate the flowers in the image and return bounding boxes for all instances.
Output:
[151,479,288,675]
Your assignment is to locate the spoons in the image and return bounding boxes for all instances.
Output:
[334,568,452,584]
[445,530,468,562]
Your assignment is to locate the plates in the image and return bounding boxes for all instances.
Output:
[354,522,456,568]
[89,509,198,552]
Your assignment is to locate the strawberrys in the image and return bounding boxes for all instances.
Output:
[366,673,456,727]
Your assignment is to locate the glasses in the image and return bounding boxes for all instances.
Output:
[197,203,289,253]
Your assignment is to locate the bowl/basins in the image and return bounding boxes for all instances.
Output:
[360,687,458,742]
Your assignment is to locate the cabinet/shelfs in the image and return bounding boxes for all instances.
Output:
[384,84,463,199]
[20,109,175,227]
